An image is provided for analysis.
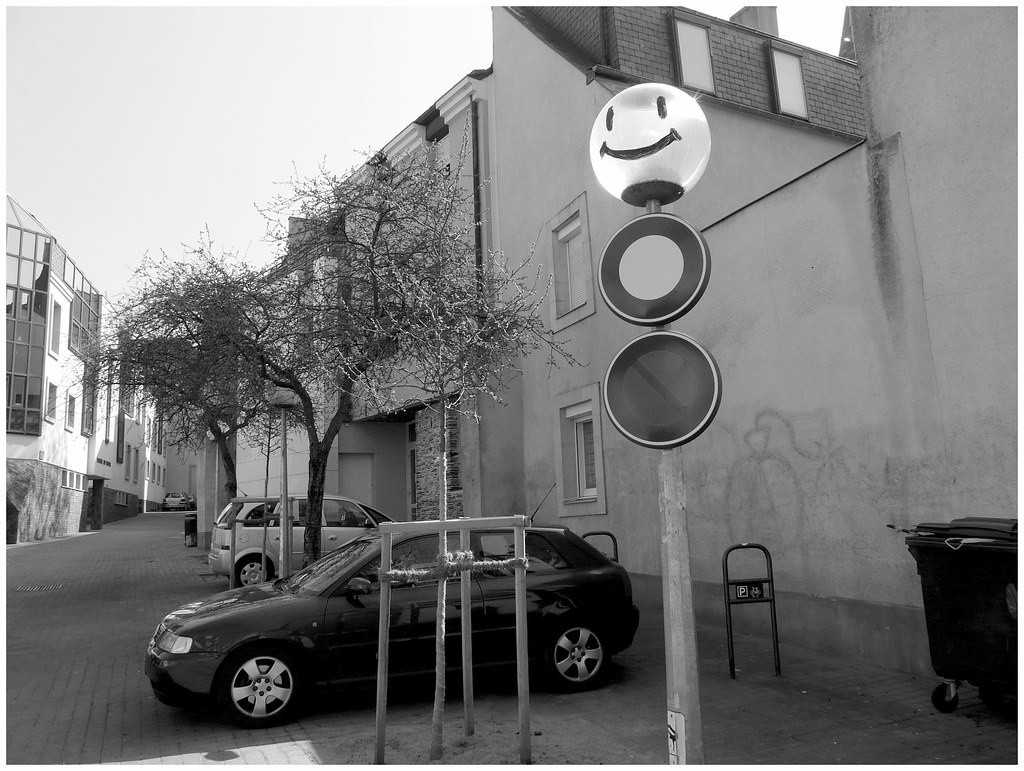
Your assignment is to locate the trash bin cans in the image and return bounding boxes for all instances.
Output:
[185,513,197,547]
[903,515,1018,729]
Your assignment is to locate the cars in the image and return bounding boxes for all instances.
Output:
[186,493,196,511]
[143,521,639,732]
[207,493,400,589]
[161,492,190,511]
